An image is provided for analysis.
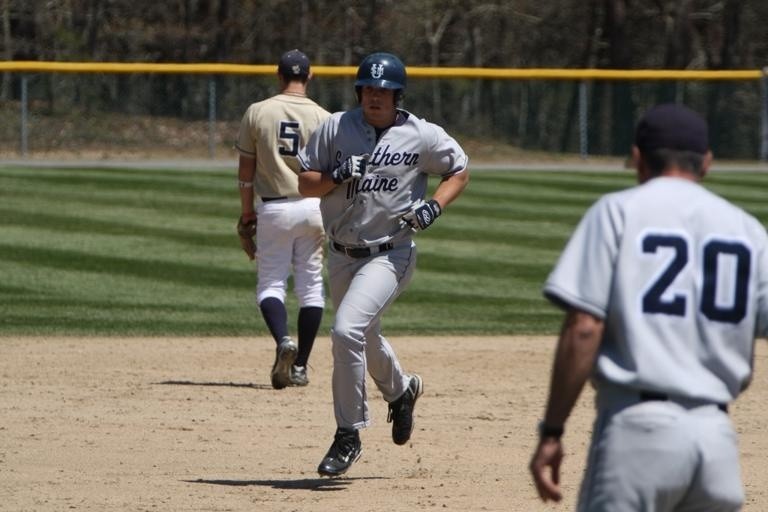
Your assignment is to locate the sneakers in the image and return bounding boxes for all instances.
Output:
[289,363,310,387]
[269,336,298,392]
[385,371,425,447]
[316,427,366,479]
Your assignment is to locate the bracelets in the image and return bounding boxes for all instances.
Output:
[538,422,567,442]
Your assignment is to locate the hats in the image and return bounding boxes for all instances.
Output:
[631,100,711,155]
[278,48,311,75]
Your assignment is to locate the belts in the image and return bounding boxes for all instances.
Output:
[260,194,288,205]
[636,387,732,418]
[331,238,393,259]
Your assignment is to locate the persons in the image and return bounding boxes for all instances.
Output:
[526,102,768,511]
[236,49,325,388]
[297,51,473,476]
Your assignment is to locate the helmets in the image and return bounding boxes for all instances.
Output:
[353,51,409,92]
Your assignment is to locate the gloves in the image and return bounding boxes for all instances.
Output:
[329,154,371,186]
[400,198,442,232]
